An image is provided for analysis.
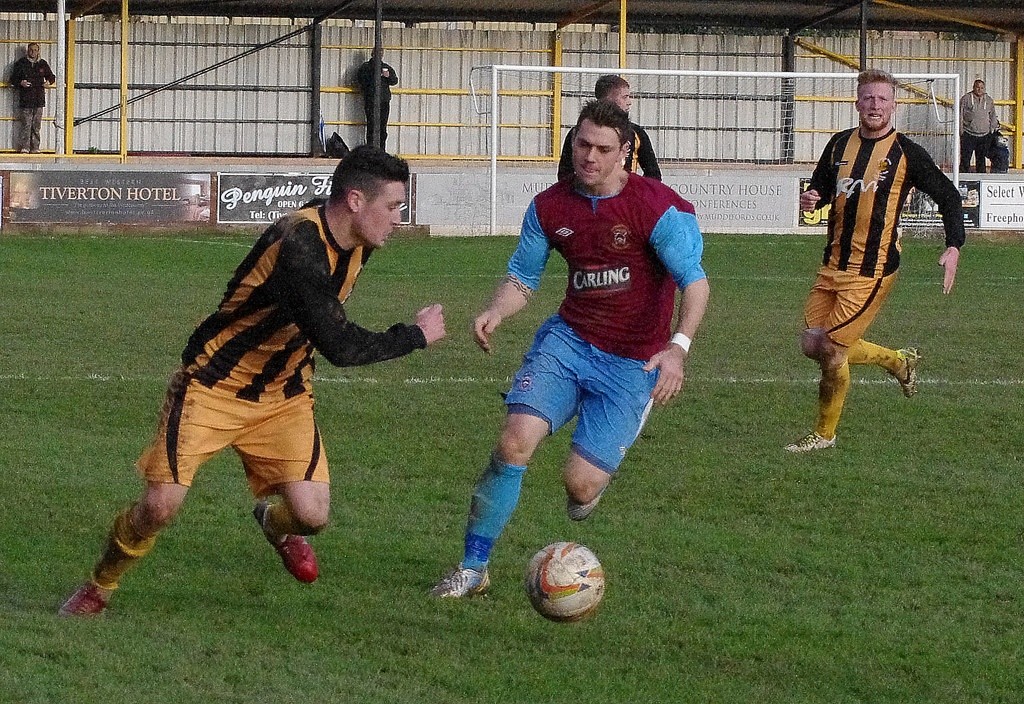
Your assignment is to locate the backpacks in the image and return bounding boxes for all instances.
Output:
[325,132,348,158]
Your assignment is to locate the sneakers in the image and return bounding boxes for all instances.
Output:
[567,486,606,521]
[58,574,117,617]
[784,431,836,452]
[895,347,919,397]
[254,503,317,583]
[21,148,40,154]
[434,563,490,598]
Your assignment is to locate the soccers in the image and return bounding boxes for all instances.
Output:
[525,542,606,622]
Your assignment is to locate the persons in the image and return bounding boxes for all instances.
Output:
[959,80,997,173]
[12,43,55,154]
[424,97,709,600]
[556,75,663,182]
[59,144,445,615]
[359,48,398,151]
[784,69,964,451]
